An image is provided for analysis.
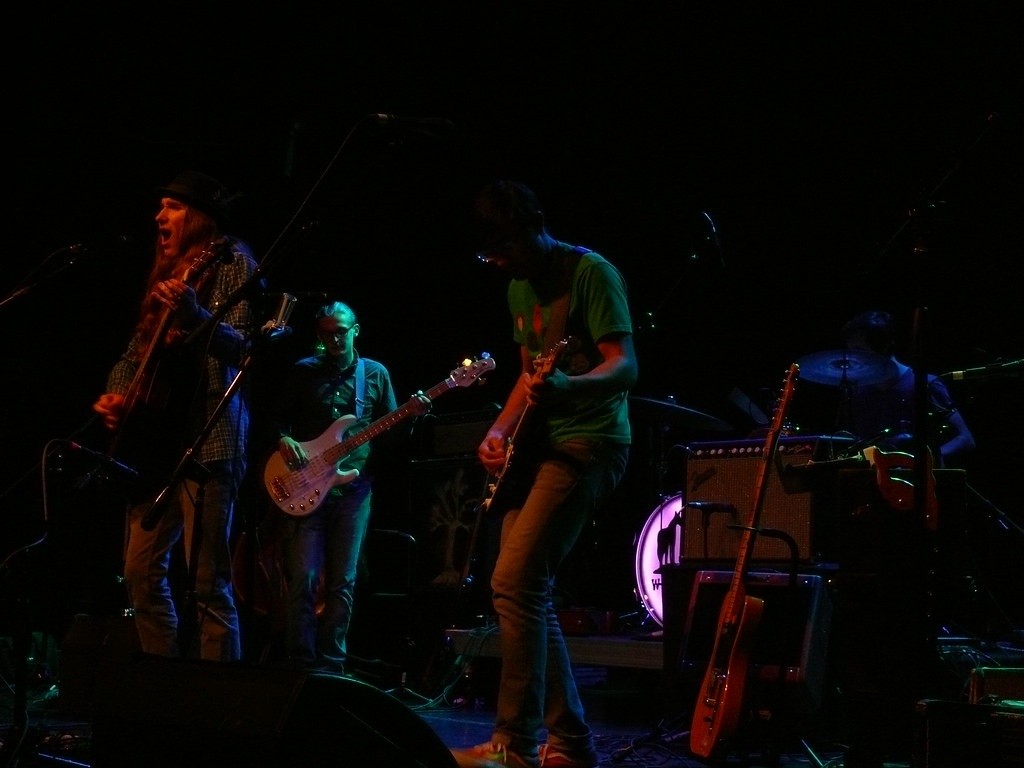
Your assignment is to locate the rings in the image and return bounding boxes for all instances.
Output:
[289,463,294,466]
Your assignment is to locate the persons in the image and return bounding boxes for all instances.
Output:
[785,308,976,484]
[88,169,261,663]
[446,177,639,768]
[261,300,433,680]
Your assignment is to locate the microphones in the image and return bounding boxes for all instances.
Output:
[688,503,734,513]
[67,440,139,478]
[68,235,127,253]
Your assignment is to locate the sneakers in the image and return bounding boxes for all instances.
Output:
[537,743,598,768]
[449,740,533,768]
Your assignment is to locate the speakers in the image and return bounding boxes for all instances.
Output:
[684,437,859,567]
[679,571,833,682]
[91,654,460,768]
[409,455,536,594]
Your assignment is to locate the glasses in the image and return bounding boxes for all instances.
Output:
[475,241,525,262]
[322,324,356,341]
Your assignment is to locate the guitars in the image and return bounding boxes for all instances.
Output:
[485,334,573,512]
[119,237,227,414]
[264,352,496,517]
[689,361,800,760]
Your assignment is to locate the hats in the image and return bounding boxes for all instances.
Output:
[152,172,229,225]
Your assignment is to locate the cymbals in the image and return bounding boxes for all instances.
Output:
[633,392,722,426]
